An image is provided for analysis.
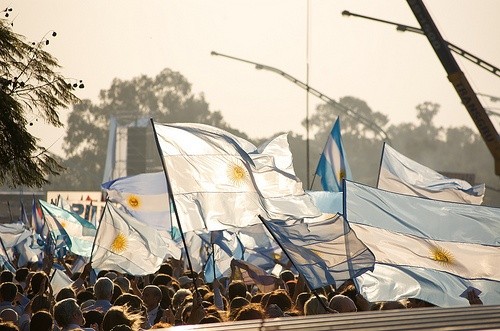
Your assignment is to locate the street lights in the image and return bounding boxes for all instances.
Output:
[210,51,390,145]
[341,10,500,80]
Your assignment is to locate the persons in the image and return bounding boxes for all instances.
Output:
[1,254,483,331]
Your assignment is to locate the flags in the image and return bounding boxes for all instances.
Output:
[0,112,499,310]
